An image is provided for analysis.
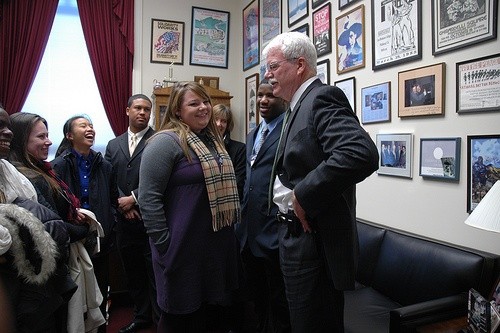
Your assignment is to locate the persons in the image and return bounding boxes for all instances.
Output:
[50,113,123,330]
[139,81,245,333]
[8,111,96,333]
[261,29,380,333]
[381,143,407,169]
[337,16,364,69]
[0,106,46,333]
[211,99,248,206]
[103,90,163,332]
[409,84,429,105]
[242,78,297,333]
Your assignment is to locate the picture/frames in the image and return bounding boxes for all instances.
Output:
[371,0,423,70]
[244,73,259,143]
[338,0,359,10]
[242,0,261,71]
[488,302,500,333]
[334,77,356,116]
[456,53,500,114]
[467,134,500,215]
[316,58,330,86]
[398,62,446,117]
[312,2,332,57]
[312,0,328,8]
[464,288,491,333]
[258,0,283,86]
[375,133,414,179]
[490,281,500,317]
[286,0,309,28]
[335,4,365,75]
[290,23,309,38]
[418,137,462,180]
[150,18,185,66]
[189,6,230,69]
[431,0,499,56]
[361,81,392,125]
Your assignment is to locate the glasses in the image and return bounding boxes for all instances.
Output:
[265,57,299,72]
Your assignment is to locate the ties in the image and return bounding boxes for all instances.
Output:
[249,126,272,164]
[128,134,139,158]
[267,105,293,219]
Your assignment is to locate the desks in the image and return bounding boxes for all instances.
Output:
[409,316,467,333]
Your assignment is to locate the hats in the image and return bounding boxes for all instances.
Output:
[337,20,363,47]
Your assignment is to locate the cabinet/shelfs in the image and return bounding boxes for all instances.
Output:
[152,83,234,136]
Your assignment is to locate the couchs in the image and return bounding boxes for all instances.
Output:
[340,217,500,333]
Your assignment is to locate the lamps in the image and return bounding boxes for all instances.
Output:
[464,181,500,233]
